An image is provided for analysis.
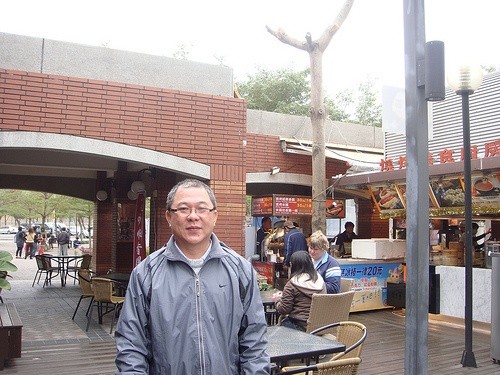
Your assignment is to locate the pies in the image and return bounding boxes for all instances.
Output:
[378,189,399,208]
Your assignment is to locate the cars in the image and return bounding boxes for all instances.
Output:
[0,222,93,239]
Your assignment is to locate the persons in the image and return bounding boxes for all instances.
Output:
[306,230,341,360]
[272,250,327,368]
[57,227,70,261]
[459,223,492,252]
[14,226,54,259]
[336,222,358,255]
[115,179,271,375]
[256,215,309,278]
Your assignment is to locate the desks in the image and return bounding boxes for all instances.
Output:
[266,327,345,375]
[105,273,130,318]
[260,290,283,309]
[43,256,85,288]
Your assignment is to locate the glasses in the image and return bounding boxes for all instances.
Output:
[169,207,215,216]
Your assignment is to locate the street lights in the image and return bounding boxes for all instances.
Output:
[446,59,480,368]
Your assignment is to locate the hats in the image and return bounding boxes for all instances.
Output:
[28,228,34,232]
[282,221,296,229]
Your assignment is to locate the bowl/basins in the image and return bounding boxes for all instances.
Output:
[474,176,495,196]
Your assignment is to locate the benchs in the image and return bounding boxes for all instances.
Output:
[0,302,23,370]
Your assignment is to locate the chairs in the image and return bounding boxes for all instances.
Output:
[280,278,367,375]
[31,253,125,335]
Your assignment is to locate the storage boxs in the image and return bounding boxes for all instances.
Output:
[351,239,404,259]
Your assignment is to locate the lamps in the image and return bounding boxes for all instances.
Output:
[270,166,280,176]
